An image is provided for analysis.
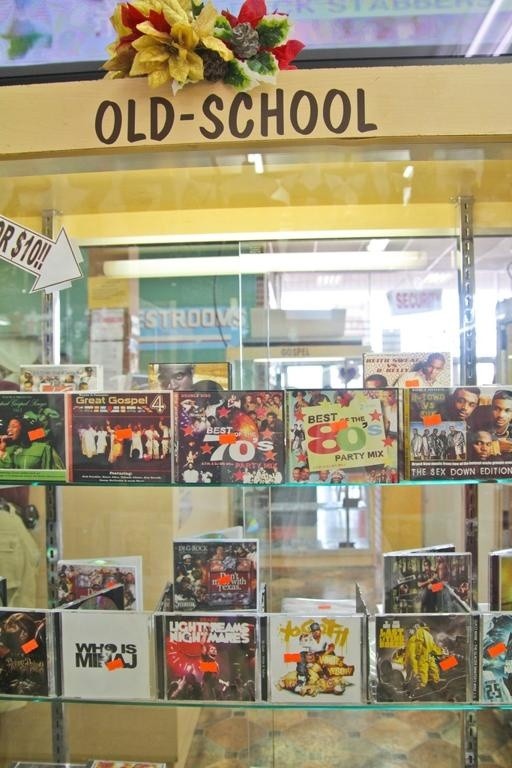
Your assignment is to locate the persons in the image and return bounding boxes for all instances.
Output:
[365,375,390,388]
[412,388,512,463]
[293,622,343,695]
[2,416,64,471]
[175,542,258,607]
[22,366,98,391]
[286,389,398,482]
[181,392,282,484]
[75,417,173,461]
[196,636,225,698]
[392,353,447,387]
[416,556,456,613]
[156,363,223,391]
[60,565,136,606]
[403,621,452,695]
[0,612,47,694]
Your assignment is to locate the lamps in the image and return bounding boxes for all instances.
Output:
[103,238,424,281]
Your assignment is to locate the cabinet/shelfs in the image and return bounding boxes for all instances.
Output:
[1,479,512,713]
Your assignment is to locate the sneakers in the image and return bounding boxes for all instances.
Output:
[294,676,307,692]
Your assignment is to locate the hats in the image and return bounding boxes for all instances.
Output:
[310,622,319,632]
